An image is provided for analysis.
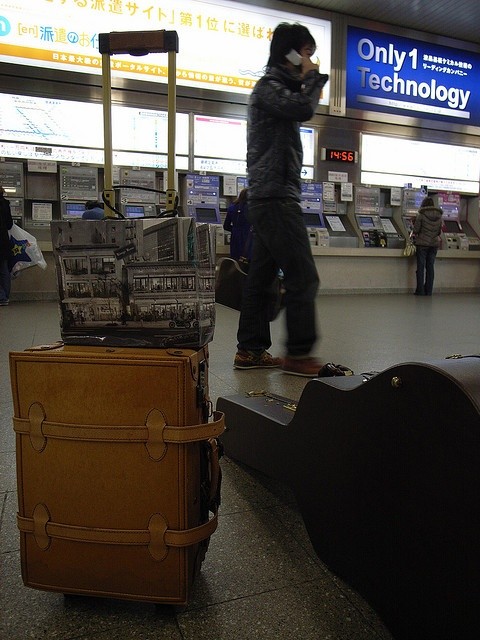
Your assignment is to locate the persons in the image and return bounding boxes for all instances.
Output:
[0,187,13,306]
[83,200,104,220]
[408,198,443,296]
[232,21,329,377]
[223,189,253,265]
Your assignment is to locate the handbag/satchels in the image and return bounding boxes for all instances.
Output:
[215,254,287,324]
[403,230,417,257]
[51,186,215,347]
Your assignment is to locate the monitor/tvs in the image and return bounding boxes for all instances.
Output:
[359,217,375,226]
[196,208,218,223]
[444,220,460,233]
[125,206,144,218]
[66,203,86,219]
[302,213,323,226]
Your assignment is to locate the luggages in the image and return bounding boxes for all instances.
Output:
[10,27,225,616]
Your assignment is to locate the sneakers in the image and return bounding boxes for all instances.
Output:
[234,348,283,370]
[283,357,326,376]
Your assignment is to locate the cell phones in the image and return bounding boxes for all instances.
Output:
[285,49,302,65]
